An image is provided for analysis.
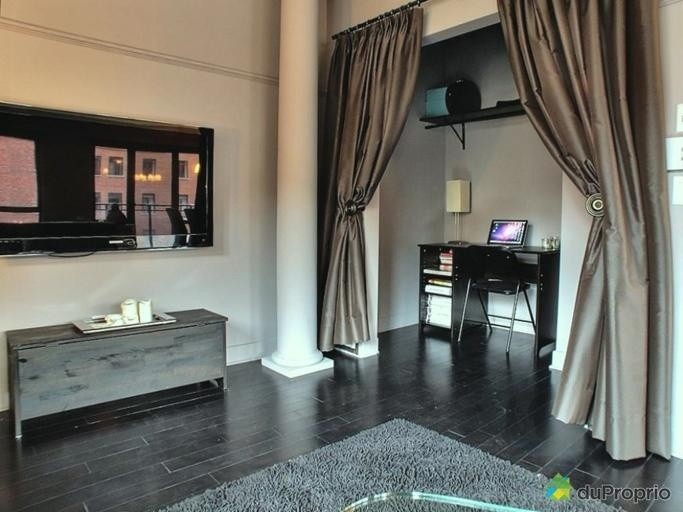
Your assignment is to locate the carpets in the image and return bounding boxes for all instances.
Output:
[147,417,625,512]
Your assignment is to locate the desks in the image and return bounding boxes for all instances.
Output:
[417,241,561,361]
[4,308,229,439]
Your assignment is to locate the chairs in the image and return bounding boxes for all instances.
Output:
[457,244,535,352]
[165,207,203,235]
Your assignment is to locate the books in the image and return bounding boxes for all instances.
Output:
[422,248,453,329]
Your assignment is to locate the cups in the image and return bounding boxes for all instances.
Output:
[542,237,559,249]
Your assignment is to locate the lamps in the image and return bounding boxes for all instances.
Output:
[445,179,471,246]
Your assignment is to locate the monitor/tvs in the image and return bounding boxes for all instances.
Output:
[0,102,214,258]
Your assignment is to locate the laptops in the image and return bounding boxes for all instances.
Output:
[479,220,529,249]
